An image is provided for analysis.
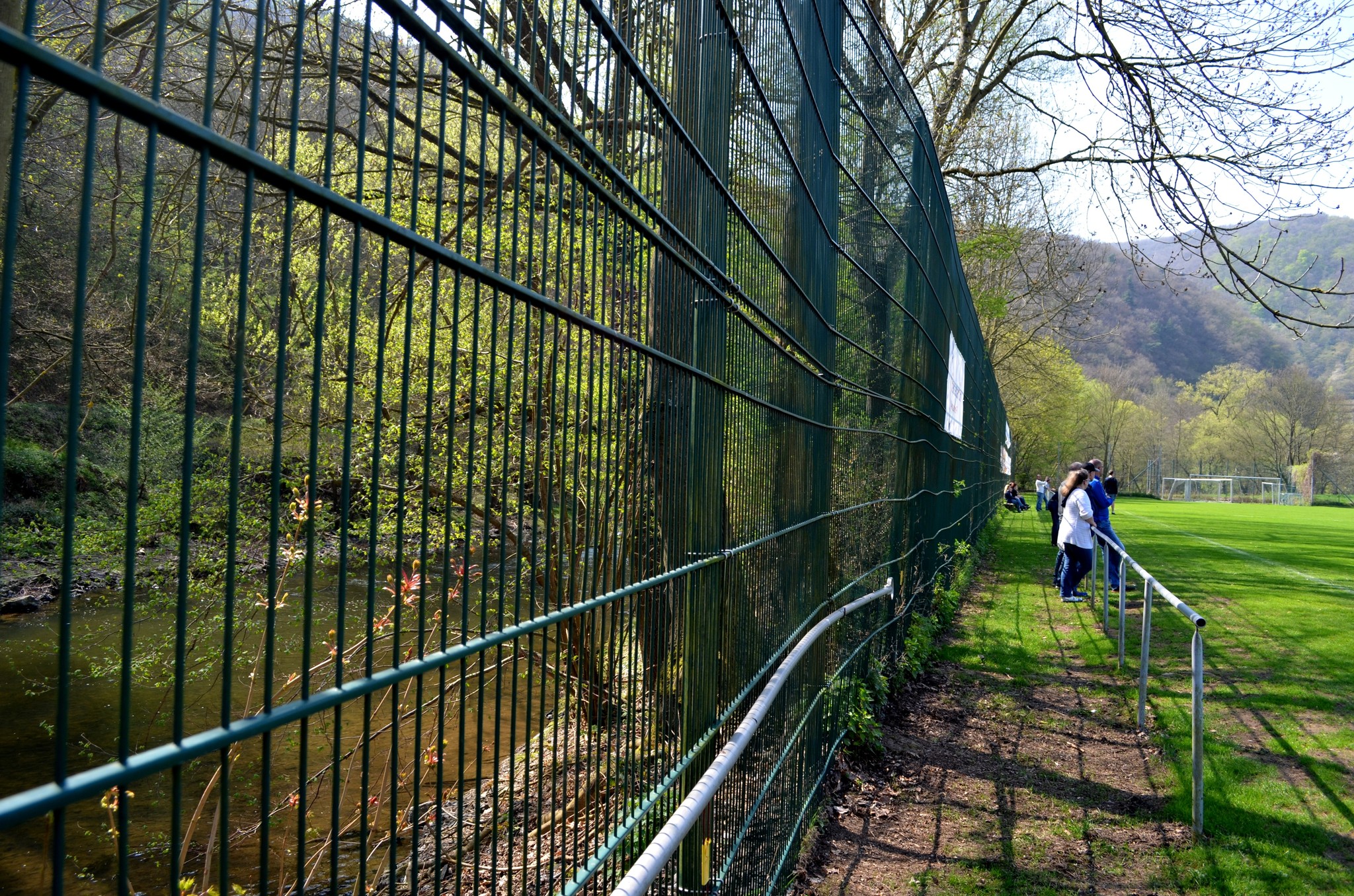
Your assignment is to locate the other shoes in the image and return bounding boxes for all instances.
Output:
[1052,544,1057,547]
[1055,585,1061,590]
[1112,513,1115,515]
[1061,596,1082,603]
[1017,504,1031,513]
[1052,580,1057,586]
[1112,585,1136,592]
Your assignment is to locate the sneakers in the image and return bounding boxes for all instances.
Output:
[1073,591,1087,597]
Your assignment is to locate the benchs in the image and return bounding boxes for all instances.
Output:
[1003,503,1015,513]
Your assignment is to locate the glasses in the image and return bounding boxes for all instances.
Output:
[1084,479,1091,482]
[1098,466,1104,469]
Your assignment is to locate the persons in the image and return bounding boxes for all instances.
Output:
[1059,469,1096,602]
[1004,460,1136,591]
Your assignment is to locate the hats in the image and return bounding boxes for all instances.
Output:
[1081,462,1100,473]
